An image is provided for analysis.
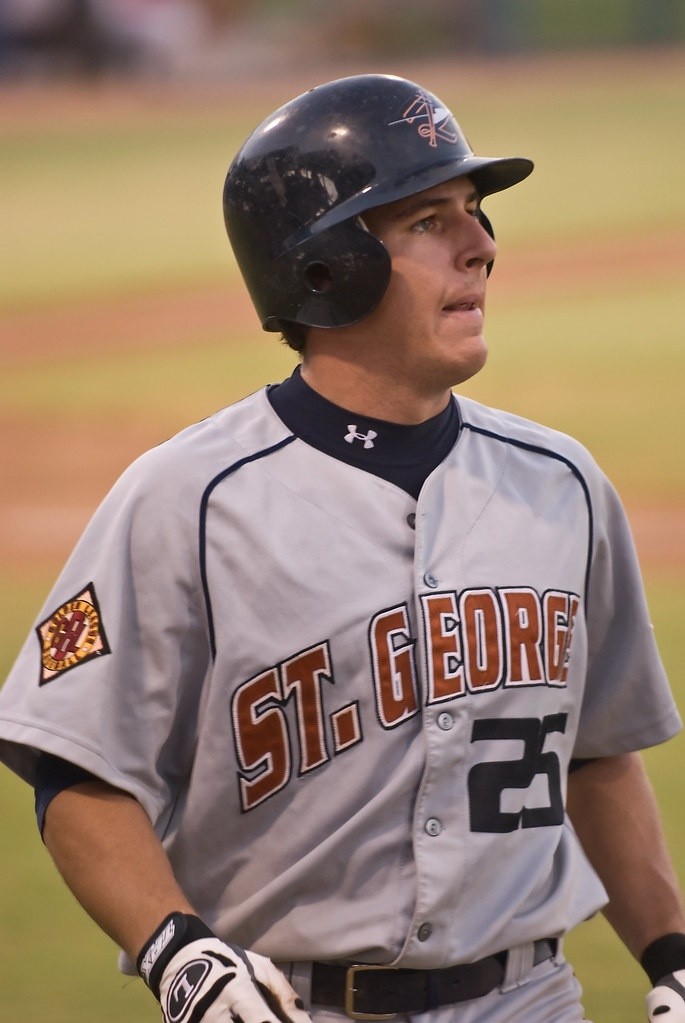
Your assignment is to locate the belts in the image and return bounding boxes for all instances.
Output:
[312,938,556,1020]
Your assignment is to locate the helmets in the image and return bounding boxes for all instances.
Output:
[222,74,535,330]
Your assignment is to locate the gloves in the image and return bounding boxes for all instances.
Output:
[642,933,685,1023]
[136,911,313,1023]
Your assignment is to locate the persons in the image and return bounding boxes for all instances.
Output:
[1,73,685,1022]
